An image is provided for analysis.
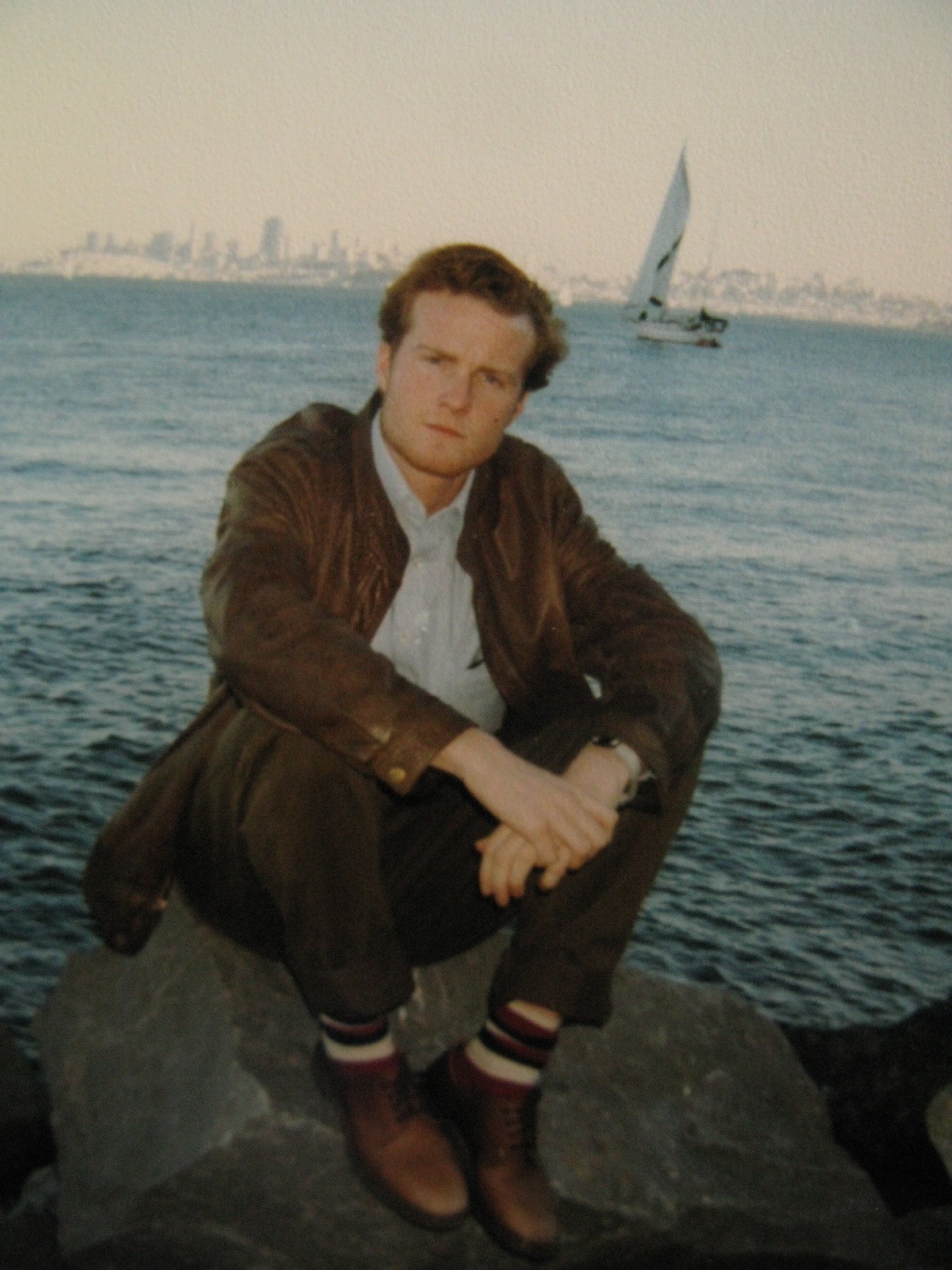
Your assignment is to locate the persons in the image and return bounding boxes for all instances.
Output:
[77,246,721,1252]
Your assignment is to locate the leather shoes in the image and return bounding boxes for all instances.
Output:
[313,1039,471,1228]
[422,1046,559,1260]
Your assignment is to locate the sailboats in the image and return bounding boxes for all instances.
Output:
[625,136,730,348]
